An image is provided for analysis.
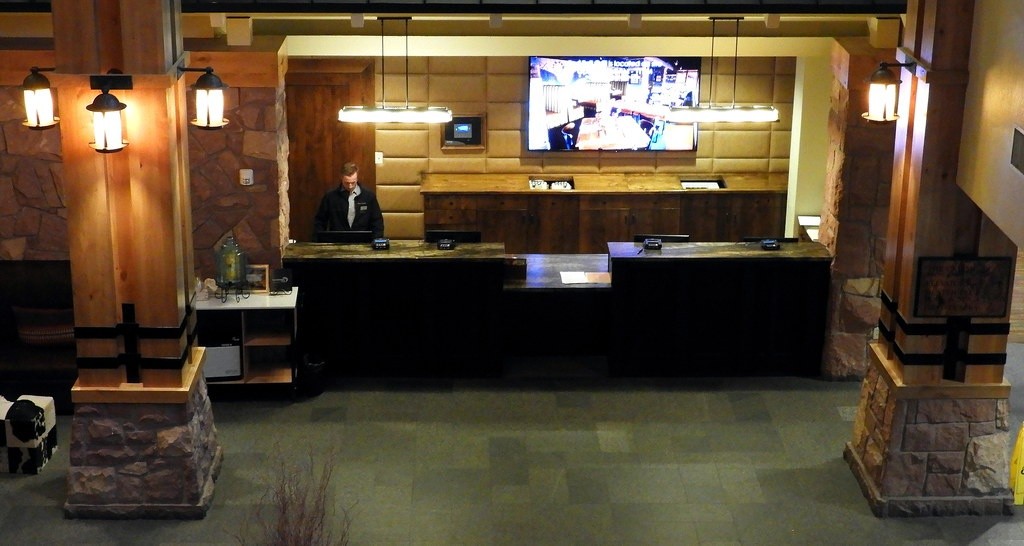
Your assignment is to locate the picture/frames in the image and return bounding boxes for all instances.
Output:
[913,256,1013,318]
[215,264,270,293]
[440,112,486,150]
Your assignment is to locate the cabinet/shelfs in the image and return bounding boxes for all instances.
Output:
[189,285,302,404]
[423,195,787,254]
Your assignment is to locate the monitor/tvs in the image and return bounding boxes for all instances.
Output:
[527,55,702,151]
[317,230,373,244]
[742,236,799,243]
[425,230,482,243]
[634,234,690,244]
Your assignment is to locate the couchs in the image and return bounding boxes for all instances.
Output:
[0,260,79,415]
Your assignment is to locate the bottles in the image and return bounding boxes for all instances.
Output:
[215,236,247,290]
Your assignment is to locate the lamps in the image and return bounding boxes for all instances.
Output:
[337,17,454,124]
[87,70,132,153]
[19,65,60,129]
[667,15,782,125]
[860,61,916,125]
[179,66,231,129]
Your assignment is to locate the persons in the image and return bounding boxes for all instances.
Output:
[310,162,385,243]
